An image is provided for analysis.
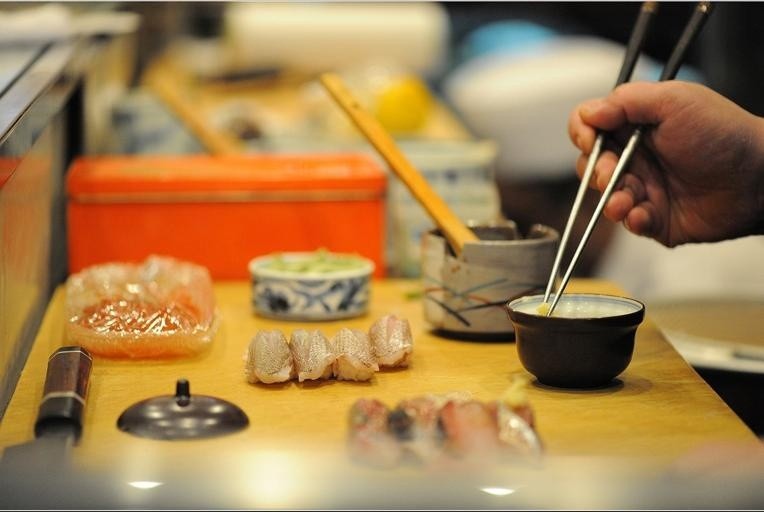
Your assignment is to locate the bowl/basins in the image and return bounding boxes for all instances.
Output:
[250,254,374,323]
[504,291,644,383]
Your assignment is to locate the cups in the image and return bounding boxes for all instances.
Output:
[418,220,560,338]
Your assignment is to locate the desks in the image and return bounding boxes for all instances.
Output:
[0,277,764,456]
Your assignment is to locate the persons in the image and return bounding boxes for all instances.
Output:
[567,79,763,248]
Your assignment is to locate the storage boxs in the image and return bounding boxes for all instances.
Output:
[67,152,387,280]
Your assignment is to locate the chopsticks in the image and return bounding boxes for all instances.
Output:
[540,1,710,313]
